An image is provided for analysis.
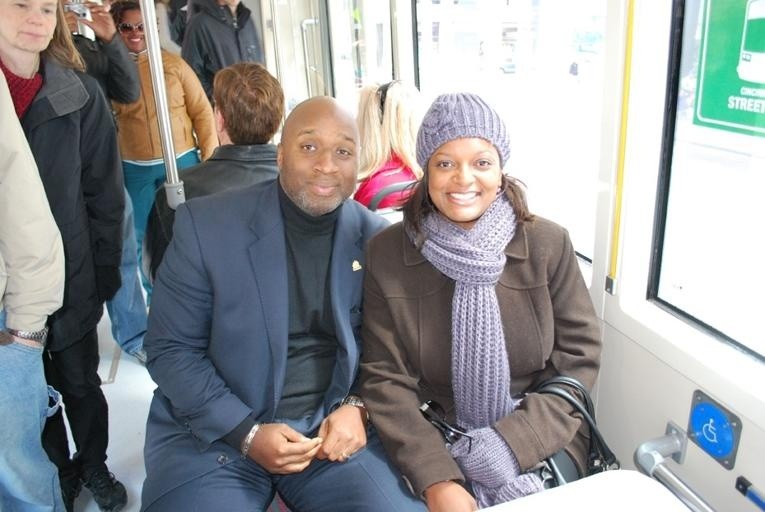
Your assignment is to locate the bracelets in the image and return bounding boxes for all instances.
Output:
[242,424,259,455]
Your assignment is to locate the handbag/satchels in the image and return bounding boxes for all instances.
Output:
[535,376,620,488]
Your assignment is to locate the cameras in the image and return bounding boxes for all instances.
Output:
[64,2,87,20]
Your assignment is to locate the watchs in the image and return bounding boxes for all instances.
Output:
[341,397,373,421]
[10,327,49,344]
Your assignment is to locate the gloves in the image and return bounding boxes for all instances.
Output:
[448,425,522,488]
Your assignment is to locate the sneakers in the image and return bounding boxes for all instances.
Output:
[80,461,129,512]
[61,463,82,512]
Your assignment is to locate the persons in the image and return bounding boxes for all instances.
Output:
[59,0,151,367]
[0,66,67,510]
[360,90,602,512]
[181,1,265,103]
[145,60,285,286]
[108,0,218,300]
[0,0,127,510]
[353,79,428,209]
[142,96,429,512]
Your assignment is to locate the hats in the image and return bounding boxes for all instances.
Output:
[414,92,512,171]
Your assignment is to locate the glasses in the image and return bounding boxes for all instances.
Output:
[376,79,400,114]
[117,22,144,35]
[419,400,475,445]
